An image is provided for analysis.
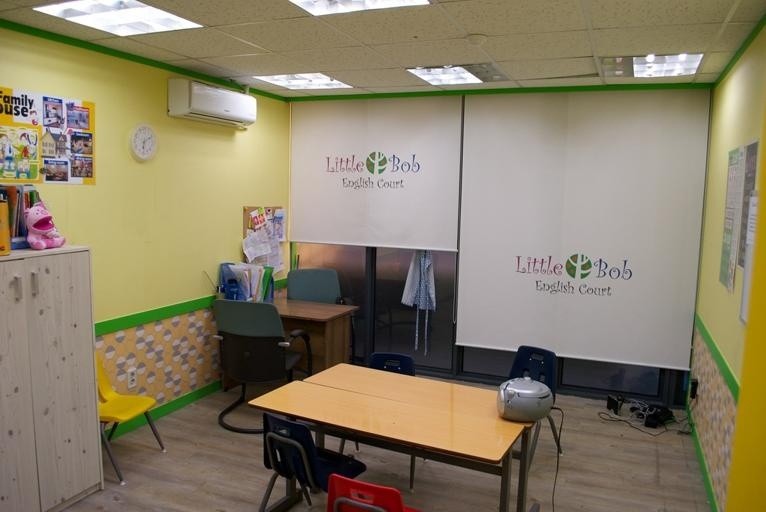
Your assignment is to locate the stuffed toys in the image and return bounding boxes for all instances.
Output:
[23,201,67,251]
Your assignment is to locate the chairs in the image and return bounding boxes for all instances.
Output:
[507,345,563,474]
[339,352,428,492]
[95,349,166,487]
[326,471,419,512]
[210,298,313,435]
[260,410,365,512]
[286,267,356,362]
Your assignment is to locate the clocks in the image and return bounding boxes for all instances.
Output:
[127,124,157,162]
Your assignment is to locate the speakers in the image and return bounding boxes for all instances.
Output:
[689,378,698,399]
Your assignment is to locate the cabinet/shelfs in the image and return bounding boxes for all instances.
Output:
[0,245,104,512]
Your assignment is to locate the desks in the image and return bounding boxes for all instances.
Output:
[275,299,359,374]
[247,364,541,512]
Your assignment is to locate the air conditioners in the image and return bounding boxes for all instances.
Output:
[166,75,257,131]
